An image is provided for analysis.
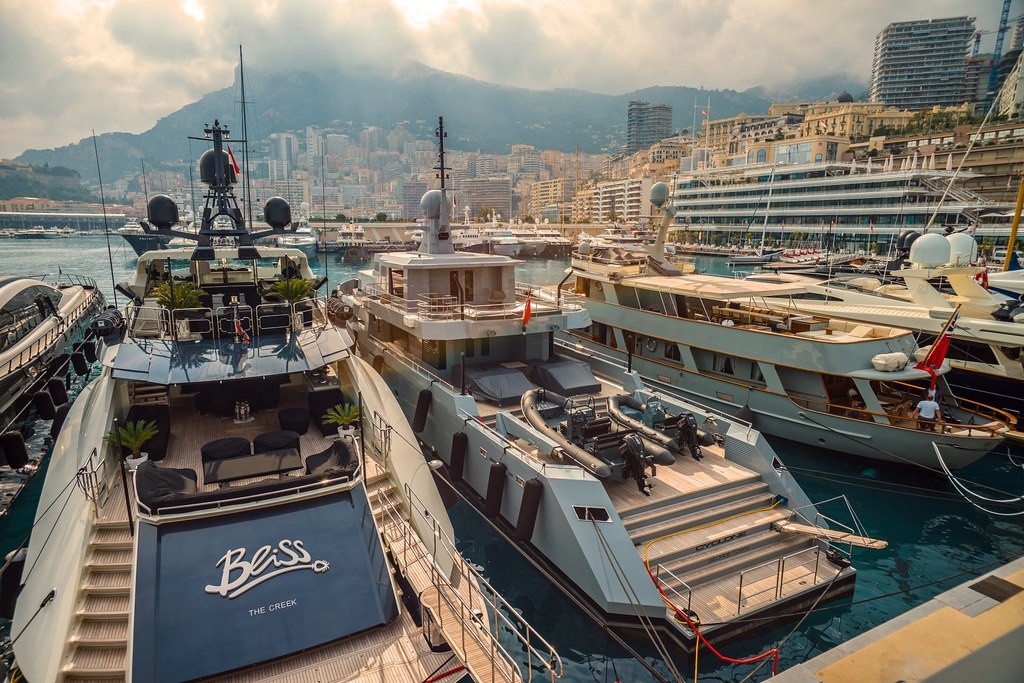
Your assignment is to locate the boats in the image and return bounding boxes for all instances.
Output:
[326,113,888,654]
[275,215,320,260]
[0,264,109,470]
[407,46,1024,480]
[118,156,240,259]
[8,42,565,683]
[334,205,366,247]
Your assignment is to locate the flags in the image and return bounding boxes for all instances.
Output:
[522,290,531,324]
[233,305,250,345]
[913,303,959,391]
[228,145,240,183]
[453,194,456,208]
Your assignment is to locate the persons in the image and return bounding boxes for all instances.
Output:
[912,382,947,432]
[975,257,988,292]
